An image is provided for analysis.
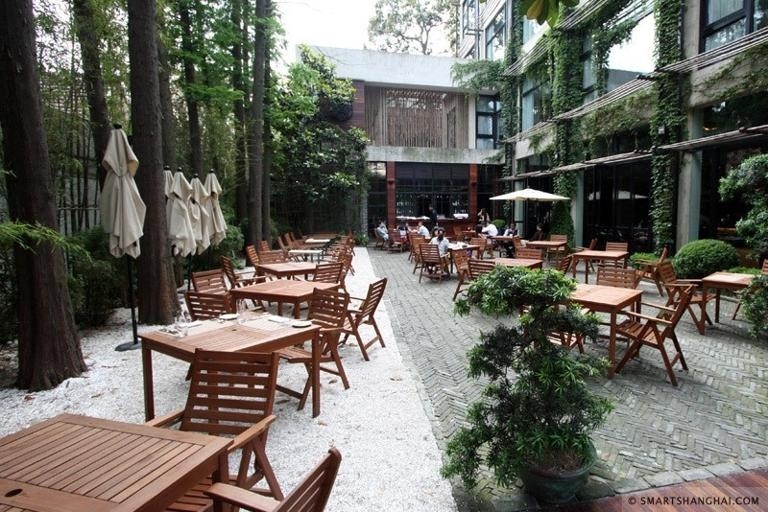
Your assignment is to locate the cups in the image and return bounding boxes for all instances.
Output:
[236,300,248,324]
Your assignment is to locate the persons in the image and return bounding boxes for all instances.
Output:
[378,203,547,260]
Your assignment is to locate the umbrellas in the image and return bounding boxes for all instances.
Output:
[489,186,569,241]
[96,125,147,352]
[162,166,228,292]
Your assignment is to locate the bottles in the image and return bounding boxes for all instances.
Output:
[174,310,191,336]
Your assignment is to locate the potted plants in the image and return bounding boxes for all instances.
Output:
[437,260,612,506]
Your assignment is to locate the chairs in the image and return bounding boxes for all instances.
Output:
[3,226,388,511]
[389,221,768,385]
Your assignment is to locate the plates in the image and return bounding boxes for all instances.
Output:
[220,314,238,319]
[292,320,312,328]
